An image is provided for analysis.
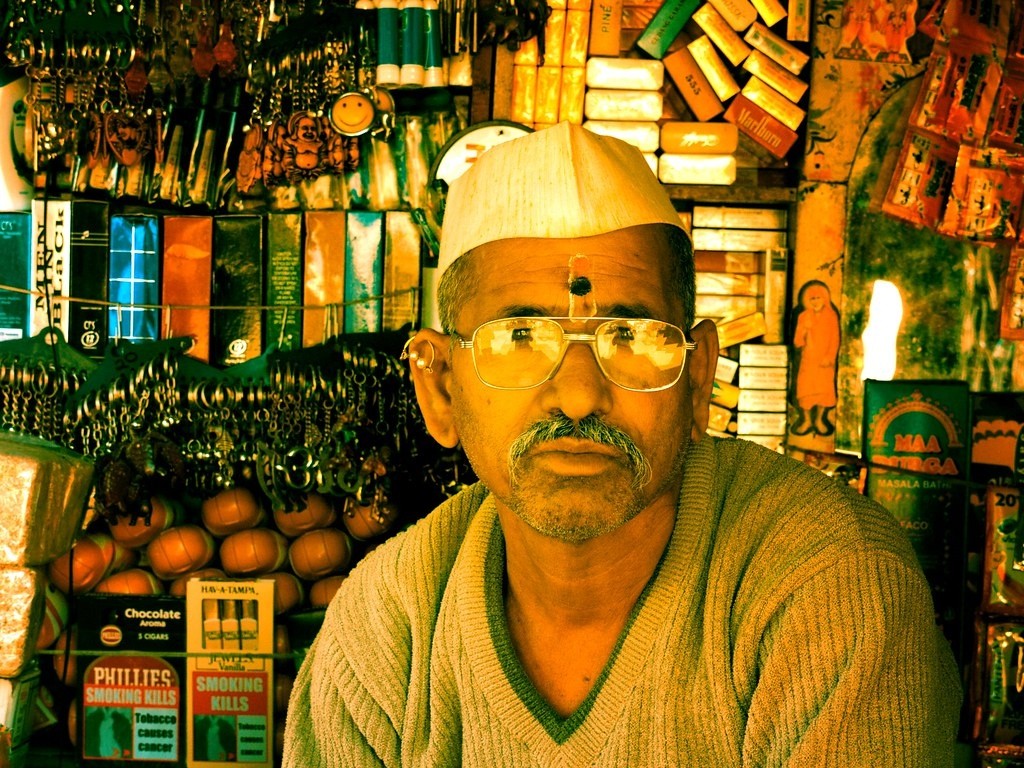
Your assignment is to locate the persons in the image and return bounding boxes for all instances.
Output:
[281,121,962,768]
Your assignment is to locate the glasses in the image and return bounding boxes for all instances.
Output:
[447,317,699,392]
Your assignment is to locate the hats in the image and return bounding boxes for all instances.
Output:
[438,120,695,289]
